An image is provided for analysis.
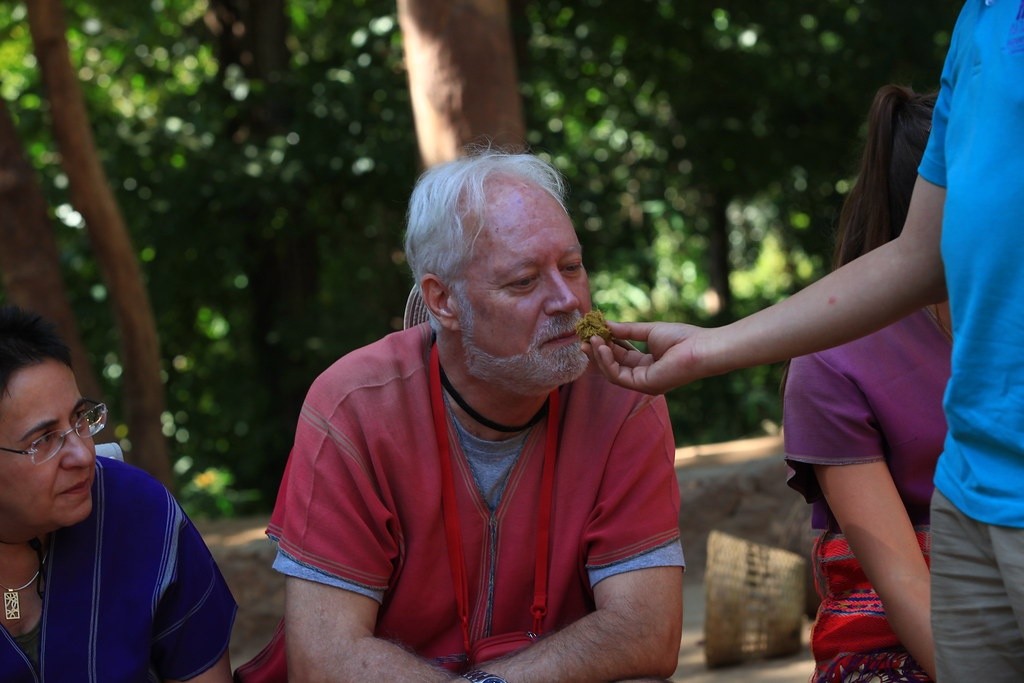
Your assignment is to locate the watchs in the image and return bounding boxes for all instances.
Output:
[462,668,509,683]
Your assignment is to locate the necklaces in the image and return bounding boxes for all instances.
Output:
[0,550,47,620]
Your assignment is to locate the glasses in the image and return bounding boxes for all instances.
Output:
[0,398,108,466]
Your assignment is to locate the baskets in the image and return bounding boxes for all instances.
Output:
[698,529,805,669]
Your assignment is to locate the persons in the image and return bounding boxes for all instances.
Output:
[0,309,237,683]
[266,152,684,682]
[577,0,1024,682]
